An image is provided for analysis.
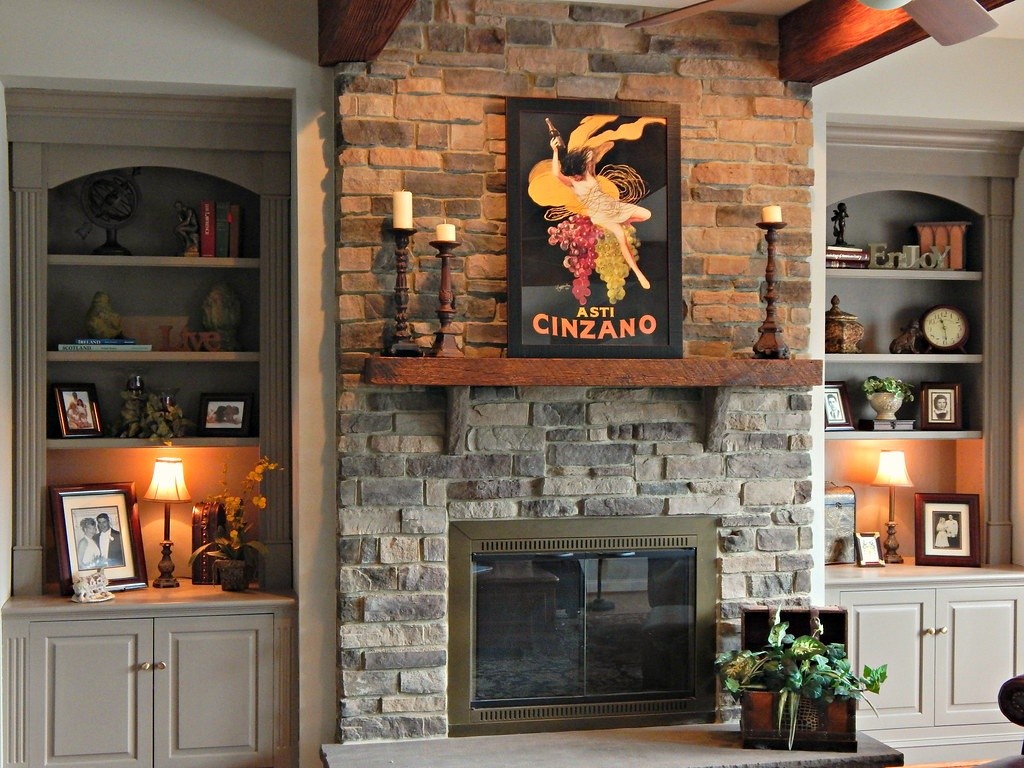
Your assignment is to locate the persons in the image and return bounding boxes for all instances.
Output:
[827,394,840,419]
[207,405,242,424]
[78,513,123,570]
[935,514,959,549]
[933,395,948,419]
[66,392,92,429]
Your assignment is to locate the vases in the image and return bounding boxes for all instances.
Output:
[215,559,248,592]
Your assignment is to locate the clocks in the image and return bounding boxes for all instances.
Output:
[918,305,971,355]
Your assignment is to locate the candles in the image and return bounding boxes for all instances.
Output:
[392,189,414,229]
[435,219,456,241]
[761,203,781,223]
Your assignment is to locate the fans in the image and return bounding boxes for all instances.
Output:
[622,0,1000,48]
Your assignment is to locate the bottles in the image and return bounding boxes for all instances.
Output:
[824,294,865,354]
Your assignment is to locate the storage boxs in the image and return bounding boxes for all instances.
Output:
[740,609,857,753]
[915,220,971,271]
[479,566,559,644]
[824,481,857,565]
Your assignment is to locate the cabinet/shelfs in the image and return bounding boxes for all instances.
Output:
[10,143,292,598]
[30,613,274,768]
[826,583,1024,728]
[825,177,1009,567]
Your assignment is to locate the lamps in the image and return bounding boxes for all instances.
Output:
[872,451,914,564]
[143,457,191,588]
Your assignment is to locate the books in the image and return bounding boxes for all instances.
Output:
[58,338,152,352]
[200,199,238,259]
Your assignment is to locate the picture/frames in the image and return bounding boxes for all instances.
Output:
[854,532,886,568]
[49,481,149,597]
[920,381,962,432]
[51,380,105,439]
[195,393,250,437]
[825,380,855,432]
[503,94,686,361]
[914,494,982,568]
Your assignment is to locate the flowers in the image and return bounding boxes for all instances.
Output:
[189,455,286,570]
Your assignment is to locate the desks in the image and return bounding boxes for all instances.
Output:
[579,552,636,612]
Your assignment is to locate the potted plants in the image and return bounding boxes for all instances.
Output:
[862,375,916,420]
